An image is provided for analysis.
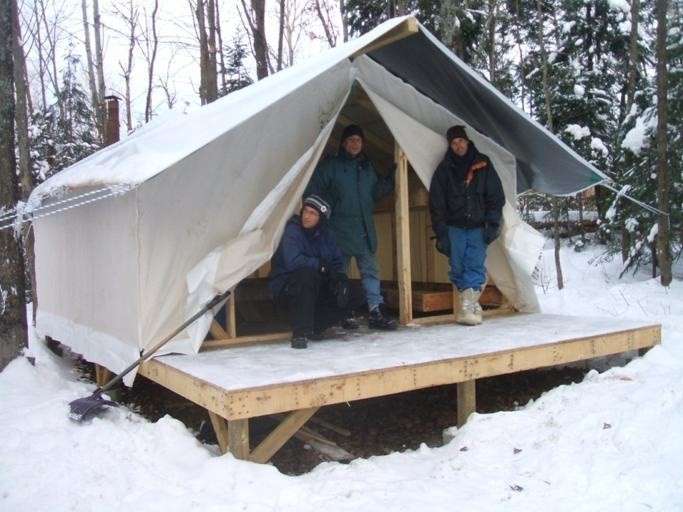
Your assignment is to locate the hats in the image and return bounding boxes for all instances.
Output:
[341,124,363,141]
[448,125,467,144]
[304,192,334,219]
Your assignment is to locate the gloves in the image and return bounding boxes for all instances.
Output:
[484,210,500,244]
[435,227,450,257]
[319,259,337,294]
[337,280,353,307]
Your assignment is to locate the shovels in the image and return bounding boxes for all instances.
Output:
[68,291,232,420]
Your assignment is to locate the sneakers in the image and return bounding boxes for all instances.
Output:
[292,310,397,348]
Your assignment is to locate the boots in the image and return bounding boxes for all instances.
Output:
[455,288,482,323]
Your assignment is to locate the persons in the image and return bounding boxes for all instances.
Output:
[428,125,506,327]
[271,190,366,349]
[301,123,404,331]
